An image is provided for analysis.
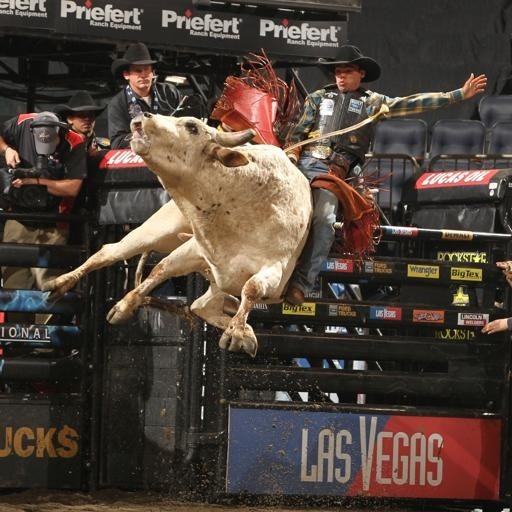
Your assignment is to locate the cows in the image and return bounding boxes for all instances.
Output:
[39,110,315,359]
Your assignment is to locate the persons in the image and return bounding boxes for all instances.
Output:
[1,109,90,357]
[107,43,206,296]
[283,44,487,306]
[54,90,112,245]
[480,258,511,511]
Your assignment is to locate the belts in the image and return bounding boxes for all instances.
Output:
[303,145,350,172]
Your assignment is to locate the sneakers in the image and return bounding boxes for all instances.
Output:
[282,285,307,309]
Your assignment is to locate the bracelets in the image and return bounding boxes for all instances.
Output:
[36,177,42,185]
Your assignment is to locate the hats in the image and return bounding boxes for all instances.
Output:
[110,43,158,77]
[52,90,109,120]
[315,44,383,83]
[31,111,60,155]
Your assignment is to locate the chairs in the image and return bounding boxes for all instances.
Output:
[362,95,512,210]
